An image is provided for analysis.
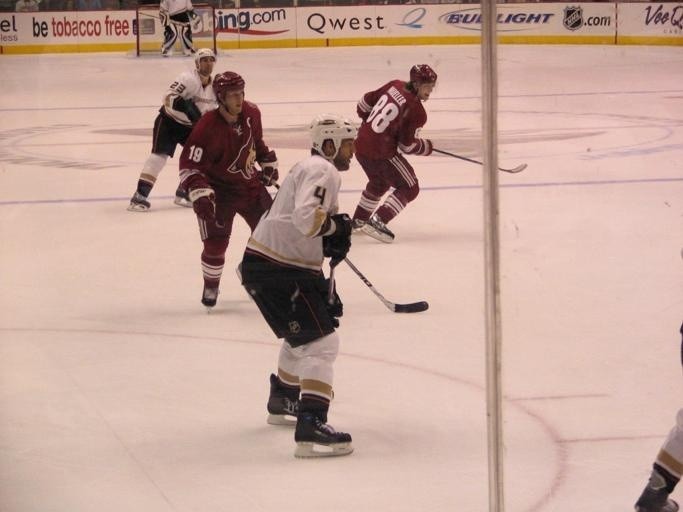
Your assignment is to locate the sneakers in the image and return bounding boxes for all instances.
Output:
[635,495,679,512]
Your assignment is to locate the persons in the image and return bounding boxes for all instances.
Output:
[156,2,197,56]
[239,114,354,444]
[348,63,435,238]
[179,72,279,304]
[633,324,683,512]
[127,47,215,206]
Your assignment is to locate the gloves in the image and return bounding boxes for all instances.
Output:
[398,135,434,159]
[171,95,201,124]
[178,173,222,225]
[255,149,282,189]
[332,211,355,240]
[321,236,353,269]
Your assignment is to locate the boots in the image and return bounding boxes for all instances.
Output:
[348,215,370,230]
[367,214,395,239]
[128,190,151,209]
[266,371,302,416]
[201,278,219,306]
[175,183,193,202]
[292,398,351,444]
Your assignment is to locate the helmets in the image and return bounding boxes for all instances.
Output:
[211,70,246,105]
[407,62,439,87]
[307,113,358,161]
[191,47,218,74]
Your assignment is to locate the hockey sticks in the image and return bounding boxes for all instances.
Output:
[269,178,429,313]
[432,148,527,174]
[139,12,201,27]
